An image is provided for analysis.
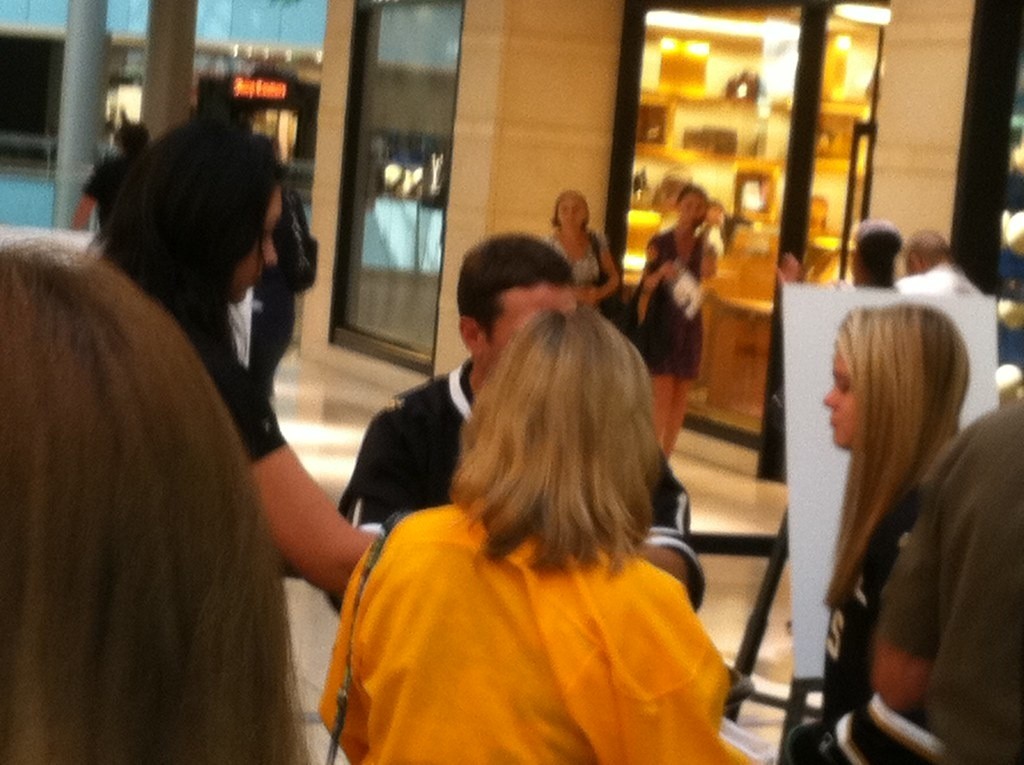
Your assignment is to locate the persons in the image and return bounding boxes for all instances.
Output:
[73,123,150,232]
[636,186,716,461]
[700,200,750,254]
[784,304,971,765]
[227,194,296,398]
[0,216,314,765]
[873,399,1024,765]
[848,219,982,297]
[93,118,375,601]
[329,232,705,619]
[319,308,761,765]
[541,190,619,307]
